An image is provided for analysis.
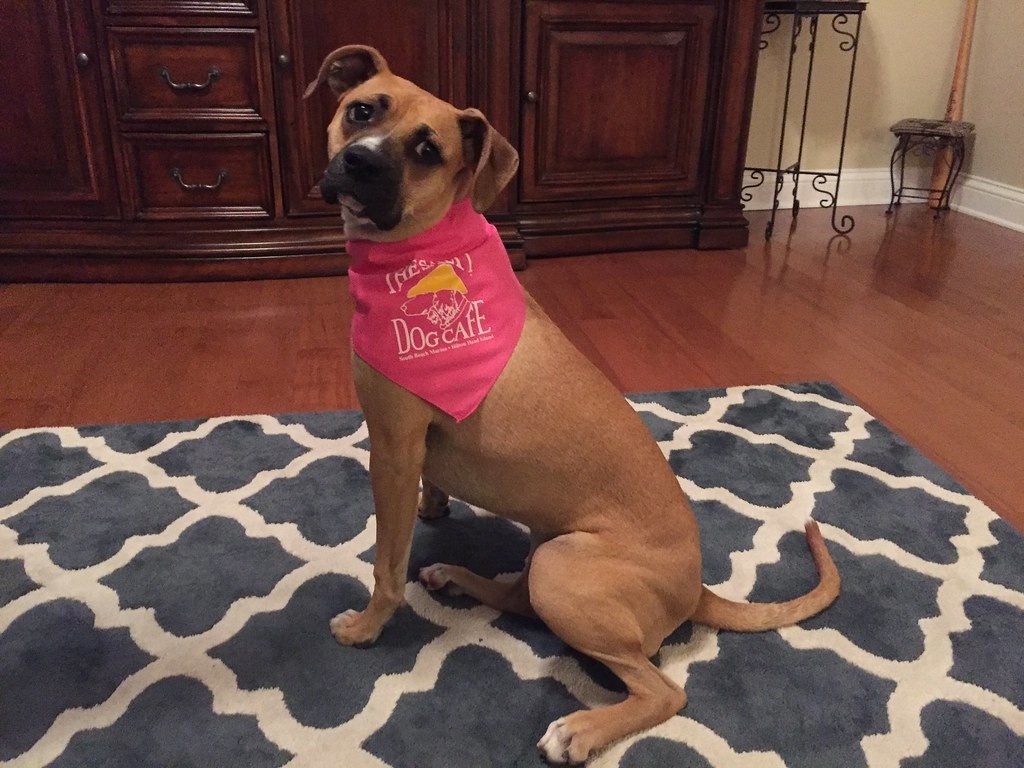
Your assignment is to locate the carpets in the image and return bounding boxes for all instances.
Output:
[1,379,1024,767]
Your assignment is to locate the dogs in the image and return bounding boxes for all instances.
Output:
[302,45,843,764]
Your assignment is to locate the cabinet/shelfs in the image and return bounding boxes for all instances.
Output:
[0,0,767,285]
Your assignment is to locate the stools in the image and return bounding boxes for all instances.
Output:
[884,117,977,220]
